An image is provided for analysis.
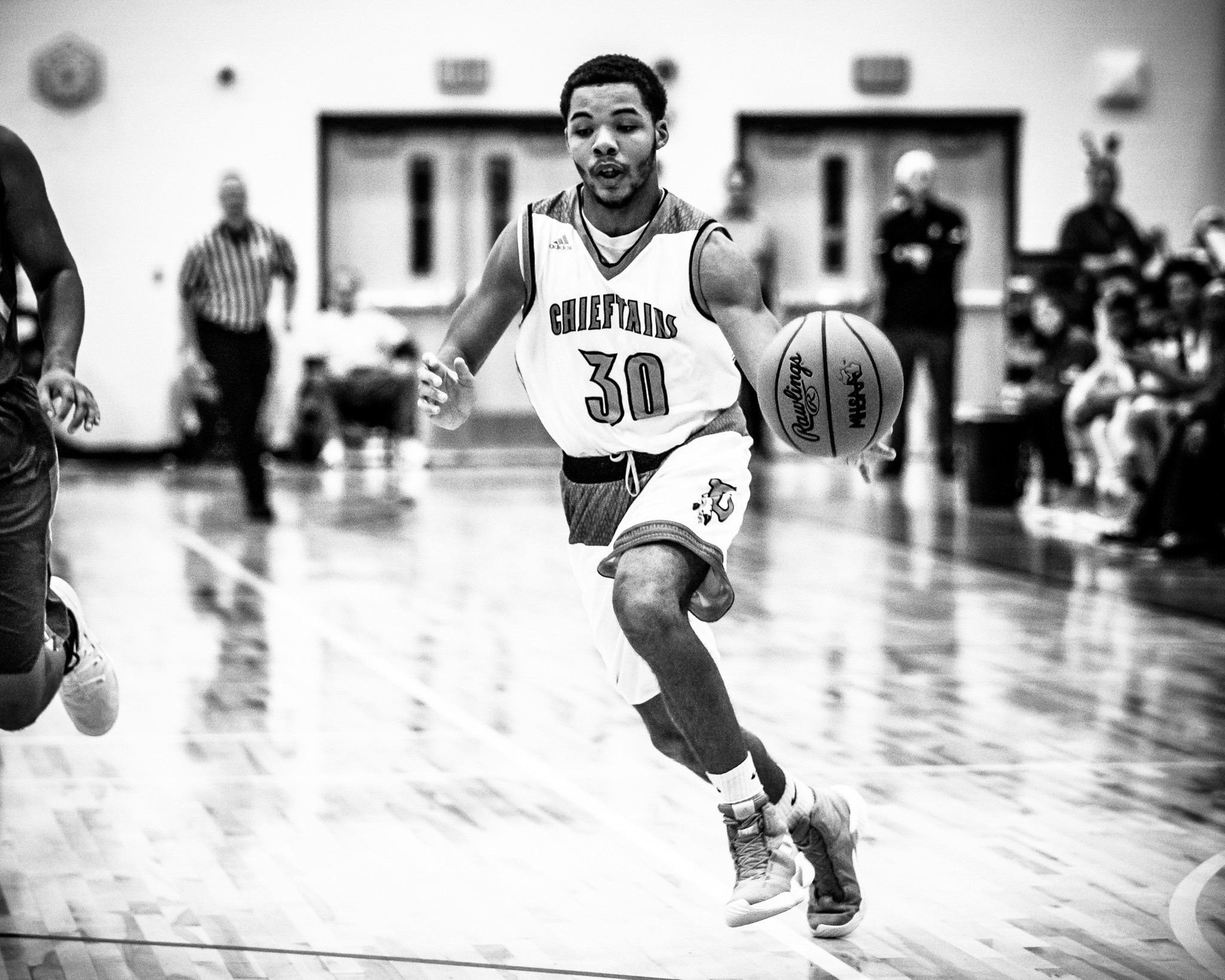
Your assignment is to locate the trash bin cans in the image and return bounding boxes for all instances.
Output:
[952,400,1032,506]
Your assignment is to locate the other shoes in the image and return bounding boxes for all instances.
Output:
[1098,526,1141,541]
[1159,532,1180,550]
[248,504,274,524]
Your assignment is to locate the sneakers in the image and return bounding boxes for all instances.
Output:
[788,786,866,939]
[718,793,816,928]
[49,576,120,737]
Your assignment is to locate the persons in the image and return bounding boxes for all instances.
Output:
[1004,157,1225,563]
[706,160,779,461]
[415,56,896,940]
[170,174,423,524]
[1,125,120,736]
[873,150,973,481]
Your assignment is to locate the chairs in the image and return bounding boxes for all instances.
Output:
[301,358,412,470]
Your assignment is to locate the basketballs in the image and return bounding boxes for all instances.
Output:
[756,310,905,458]
